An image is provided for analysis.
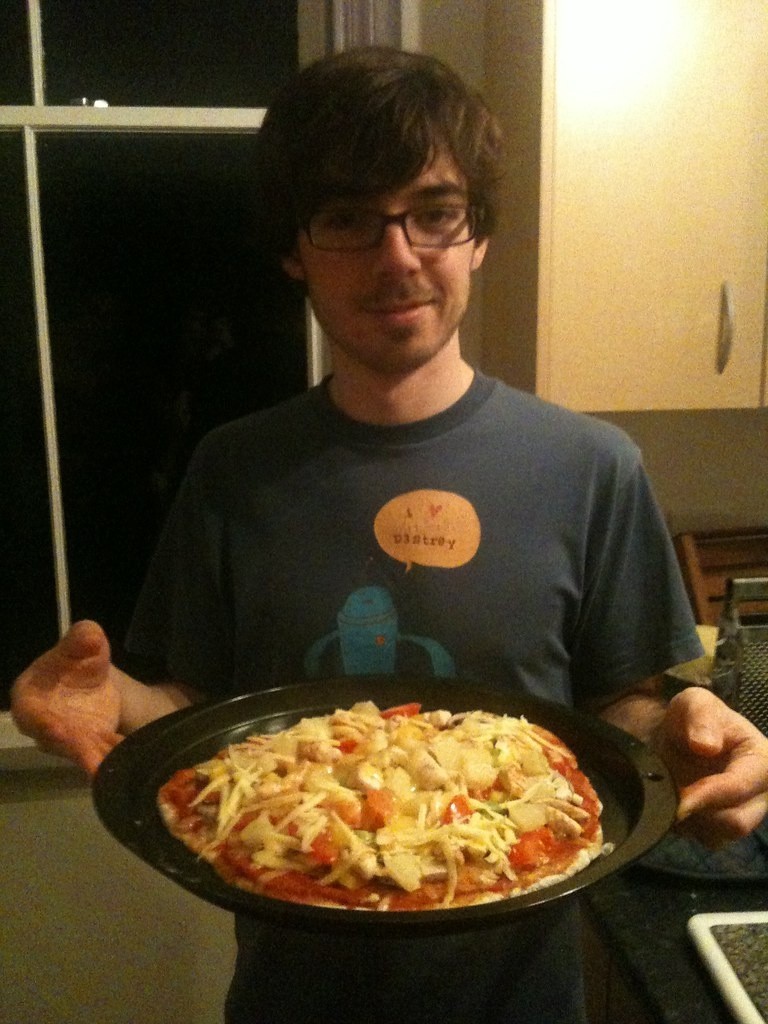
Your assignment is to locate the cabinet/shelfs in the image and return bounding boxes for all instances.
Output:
[535,0,768,413]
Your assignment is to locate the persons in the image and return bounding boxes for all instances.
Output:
[9,47,768,1024]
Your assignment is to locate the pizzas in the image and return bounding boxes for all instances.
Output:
[157,700,601,912]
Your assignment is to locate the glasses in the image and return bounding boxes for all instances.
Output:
[296,204,484,252]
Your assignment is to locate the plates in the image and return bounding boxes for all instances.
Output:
[92,675,676,934]
[638,814,768,880]
